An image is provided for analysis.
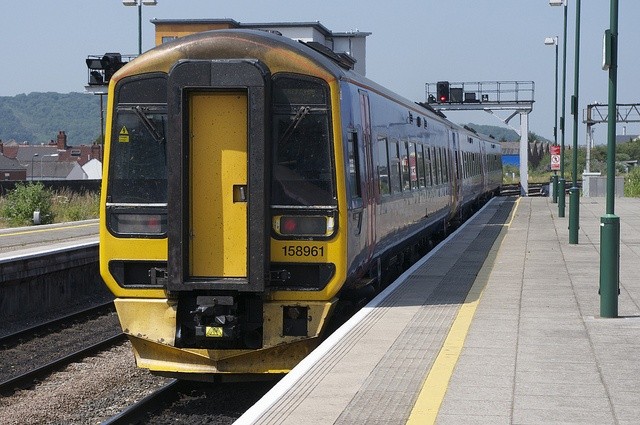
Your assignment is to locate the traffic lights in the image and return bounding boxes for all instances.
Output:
[86,53,128,86]
[428,94,436,103]
[439,83,448,102]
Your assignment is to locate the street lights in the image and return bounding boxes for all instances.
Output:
[549,0,568,217]
[123,0,158,55]
[544,35,559,204]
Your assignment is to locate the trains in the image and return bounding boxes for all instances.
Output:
[98,28,505,384]
